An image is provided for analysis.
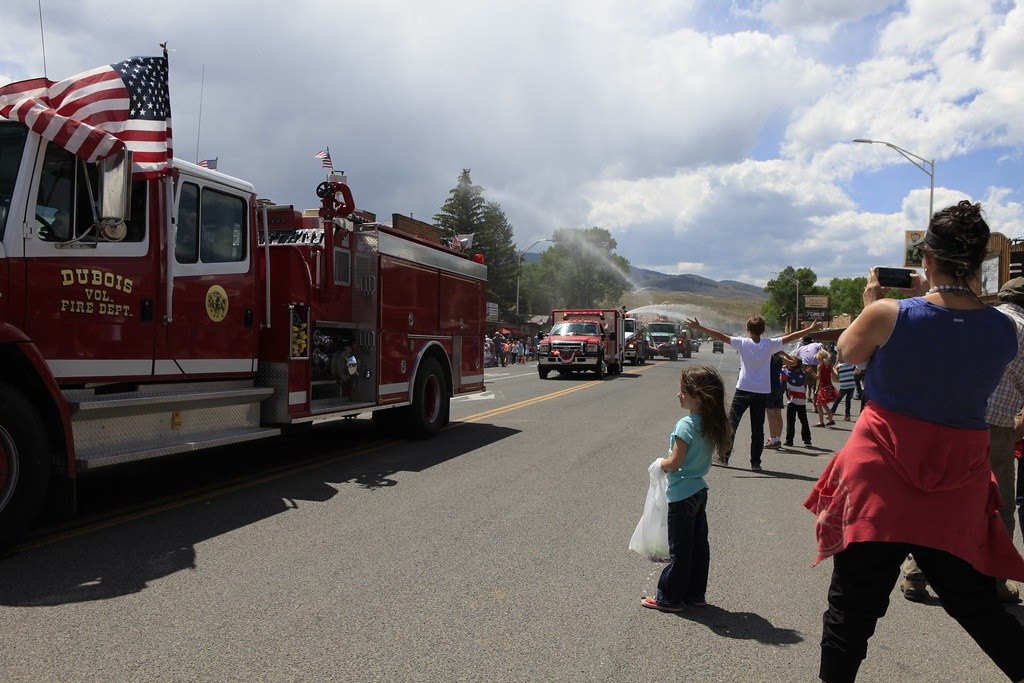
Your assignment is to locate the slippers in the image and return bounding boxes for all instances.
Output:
[642,596,683,612]
[686,599,706,606]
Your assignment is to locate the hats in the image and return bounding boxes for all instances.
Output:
[912,228,985,255]
[1000,276,1024,296]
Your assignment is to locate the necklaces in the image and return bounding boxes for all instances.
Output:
[925,286,976,296]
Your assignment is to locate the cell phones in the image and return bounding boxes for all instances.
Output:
[873,266,917,291]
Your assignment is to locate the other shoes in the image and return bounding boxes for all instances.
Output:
[783,441,793,446]
[752,465,761,471]
[826,421,835,425]
[1006,581,1019,601]
[900,578,926,601]
[714,455,728,465]
[813,423,824,427]
[804,440,812,445]
[845,417,850,421]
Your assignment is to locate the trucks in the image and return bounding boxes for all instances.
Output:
[647,321,702,361]
[713,341,724,354]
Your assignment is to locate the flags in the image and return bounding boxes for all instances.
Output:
[314,149,333,169]
[0,47,174,180]
[197,160,216,170]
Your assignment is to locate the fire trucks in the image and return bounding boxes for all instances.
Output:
[0,112,487,553]
[624,313,649,366]
[536,308,630,380]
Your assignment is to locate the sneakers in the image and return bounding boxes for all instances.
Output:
[764,438,781,449]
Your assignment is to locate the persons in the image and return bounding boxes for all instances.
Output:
[808,349,841,428]
[684,316,823,472]
[803,200,1024,683]
[763,351,797,450]
[40,168,73,241]
[619,306,628,314]
[780,357,812,446]
[795,336,838,412]
[484,329,549,368]
[641,366,733,611]
[826,355,862,421]
[854,373,863,400]
[899,277,1024,601]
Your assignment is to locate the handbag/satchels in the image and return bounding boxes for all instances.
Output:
[628,457,670,564]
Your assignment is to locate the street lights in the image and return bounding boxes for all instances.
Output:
[853,138,935,221]
[770,270,799,331]
[516,238,556,316]
